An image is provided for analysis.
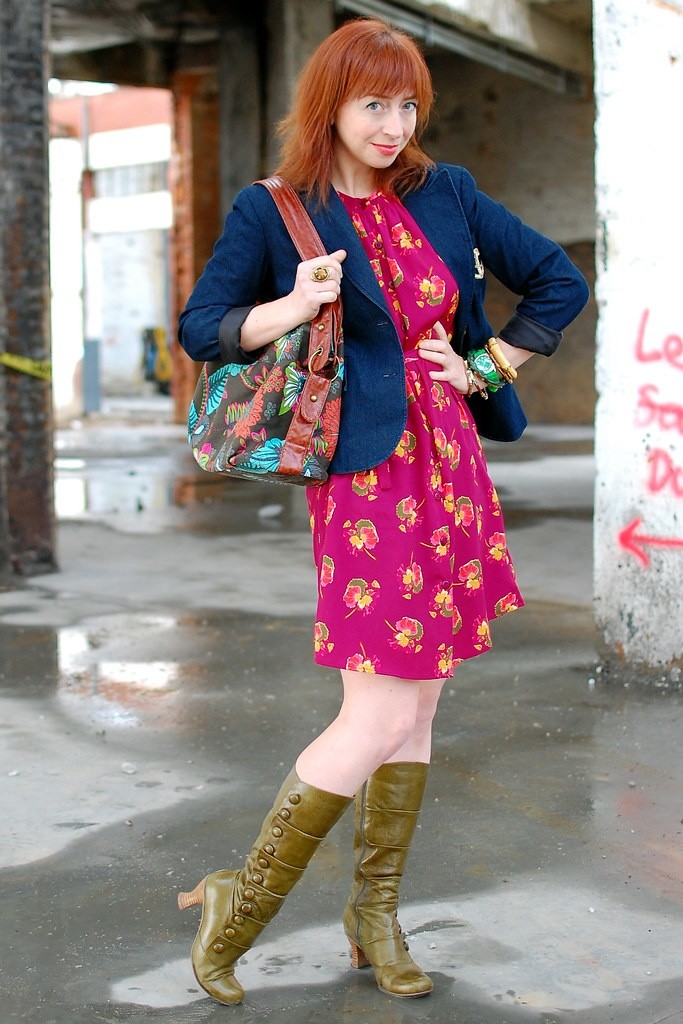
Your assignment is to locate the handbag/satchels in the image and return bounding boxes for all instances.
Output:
[186,173,345,486]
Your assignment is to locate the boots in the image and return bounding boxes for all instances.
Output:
[178,764,356,1006]
[342,760,435,998]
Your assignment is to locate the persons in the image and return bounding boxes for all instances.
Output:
[176,19,591,1004]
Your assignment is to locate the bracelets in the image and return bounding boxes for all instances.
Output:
[465,339,517,401]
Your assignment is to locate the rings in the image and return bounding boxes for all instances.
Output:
[311,266,331,282]
